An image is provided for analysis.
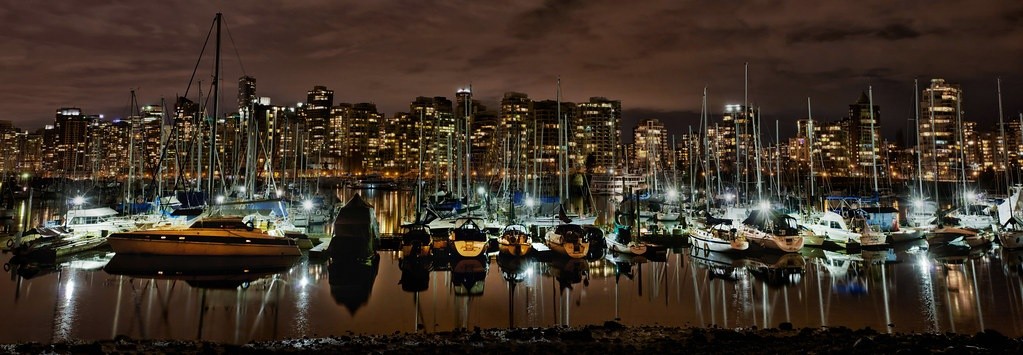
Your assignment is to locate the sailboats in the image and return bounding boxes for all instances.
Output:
[6,10,1023,277]
[3,257,1023,331]
